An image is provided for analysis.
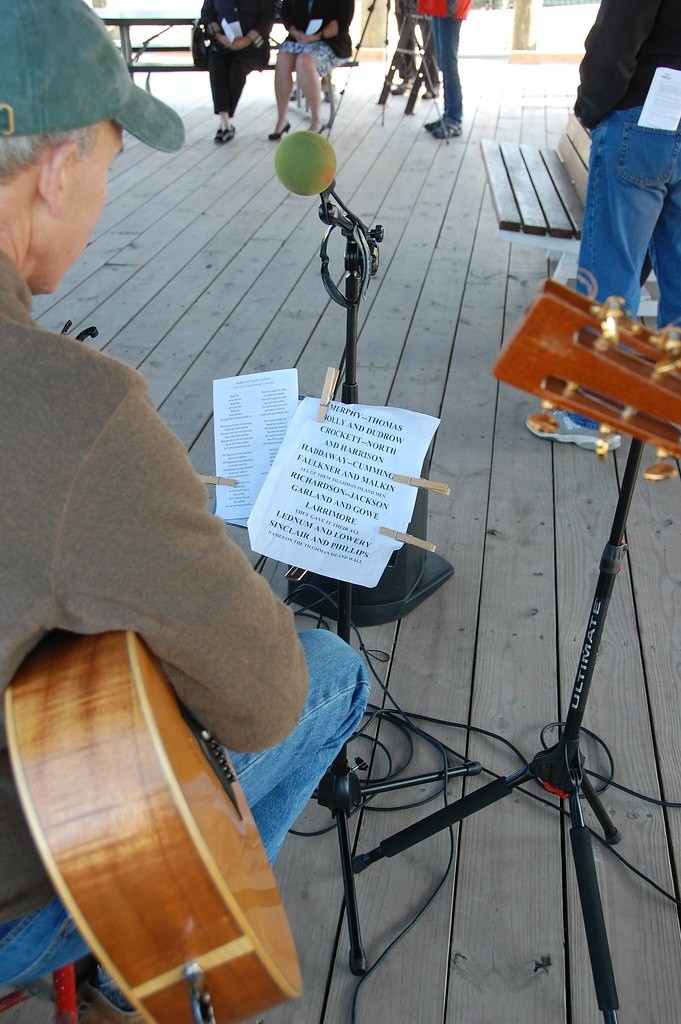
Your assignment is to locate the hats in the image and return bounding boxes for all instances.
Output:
[0,0,185,153]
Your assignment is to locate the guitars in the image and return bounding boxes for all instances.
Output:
[0,625,307,1024]
[489,286,681,455]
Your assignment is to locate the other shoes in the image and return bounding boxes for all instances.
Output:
[214,125,235,142]
[83,962,141,1014]
[390,84,407,95]
[423,89,437,99]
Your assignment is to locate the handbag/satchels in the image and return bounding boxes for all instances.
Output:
[192,20,208,69]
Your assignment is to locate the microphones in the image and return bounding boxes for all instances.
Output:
[275,130,336,195]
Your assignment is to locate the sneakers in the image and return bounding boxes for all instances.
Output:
[432,117,462,138]
[526,408,623,453]
[425,115,447,131]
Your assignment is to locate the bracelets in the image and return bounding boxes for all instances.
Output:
[318,31,324,40]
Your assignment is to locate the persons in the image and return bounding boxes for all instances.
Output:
[392,0,440,98]
[268,0,356,139]
[192,0,282,142]
[0,0,371,1024]
[527,0,681,451]
[416,0,472,138]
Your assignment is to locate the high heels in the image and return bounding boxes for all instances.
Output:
[269,122,291,140]
[309,125,325,133]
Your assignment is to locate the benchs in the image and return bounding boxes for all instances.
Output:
[478,112,659,318]
[103,17,360,130]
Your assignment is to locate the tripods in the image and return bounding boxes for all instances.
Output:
[310,197,644,1024]
[326,0,449,144]
